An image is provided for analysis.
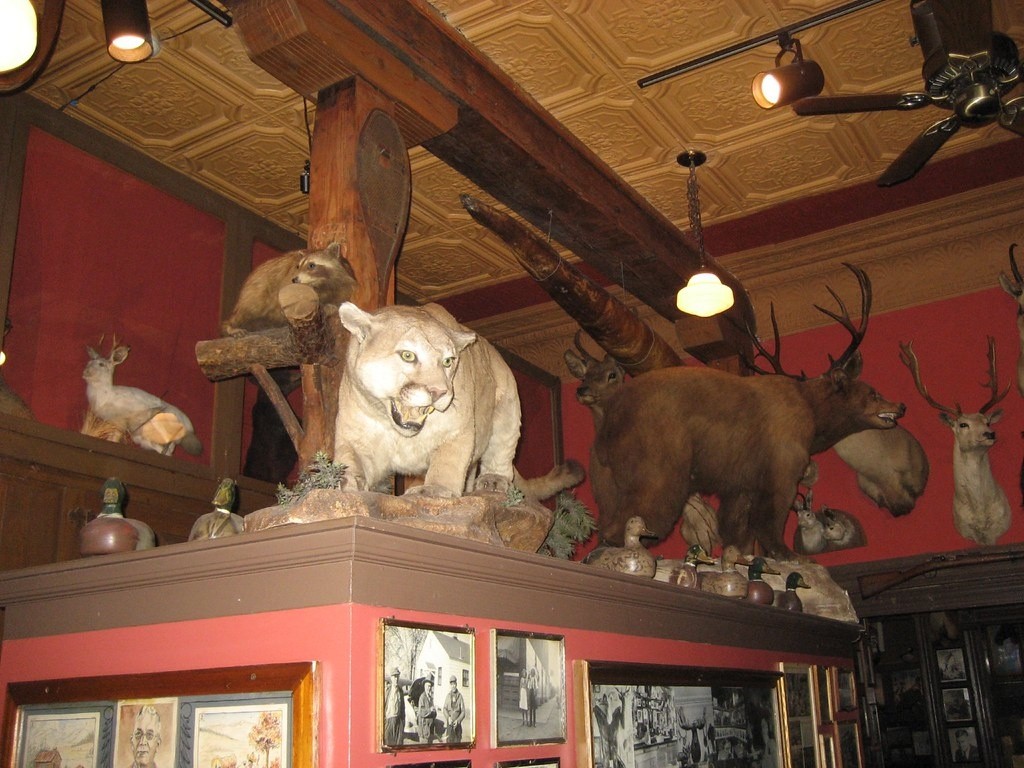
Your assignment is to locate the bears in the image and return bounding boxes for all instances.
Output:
[588,350,907,575]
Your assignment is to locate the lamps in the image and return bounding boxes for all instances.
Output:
[676,151,733,316]
[101,0,154,64]
[751,34,824,110]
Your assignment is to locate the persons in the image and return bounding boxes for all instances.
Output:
[954,730,979,761]
[443,675,466,743]
[384,667,406,746]
[416,678,437,743]
[519,667,539,727]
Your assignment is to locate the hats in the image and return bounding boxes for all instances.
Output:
[422,678,435,686]
[391,667,400,676]
[450,676,457,682]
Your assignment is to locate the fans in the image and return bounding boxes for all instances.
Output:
[794,0,1024,188]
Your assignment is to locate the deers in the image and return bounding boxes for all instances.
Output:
[720,263,929,518]
[998,243,1024,398]
[789,492,827,554]
[564,329,626,435]
[82,333,202,457]
[900,336,1013,547]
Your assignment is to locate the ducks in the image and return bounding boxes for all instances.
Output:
[695,545,754,599]
[771,572,812,613]
[652,544,718,589]
[743,559,781,605]
[580,516,657,578]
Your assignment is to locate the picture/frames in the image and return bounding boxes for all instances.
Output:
[489,628,567,748]
[833,720,863,768]
[572,659,793,768]
[779,662,821,768]
[110,694,182,768]
[819,734,836,768]
[386,759,472,768]
[865,616,984,768]
[494,757,560,768]
[832,665,858,712]
[376,617,476,753]
[23,712,100,768]
[813,665,833,725]
[193,703,288,768]
